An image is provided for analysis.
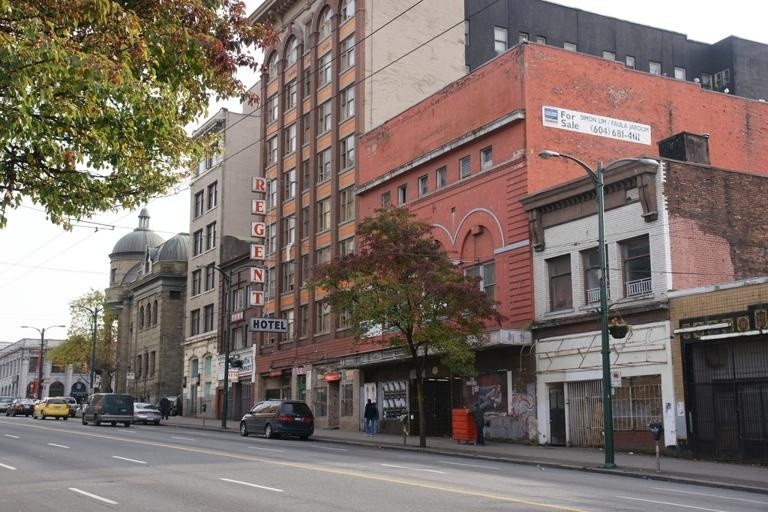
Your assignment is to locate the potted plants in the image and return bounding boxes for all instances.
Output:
[608,316,630,339]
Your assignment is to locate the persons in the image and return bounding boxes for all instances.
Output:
[470,403,485,444]
[372,402,379,434]
[159,394,170,420]
[365,399,377,435]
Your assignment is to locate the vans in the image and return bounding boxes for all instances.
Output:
[81,392,134,427]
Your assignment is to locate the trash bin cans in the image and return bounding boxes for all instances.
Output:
[452,408,478,446]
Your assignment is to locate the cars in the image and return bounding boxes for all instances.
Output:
[133,402,161,425]
[0,396,88,420]
[240,398,314,439]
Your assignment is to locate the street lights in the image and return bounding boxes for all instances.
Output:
[538,150,661,470]
[70,304,124,388]
[196,264,266,429]
[21,325,66,400]
